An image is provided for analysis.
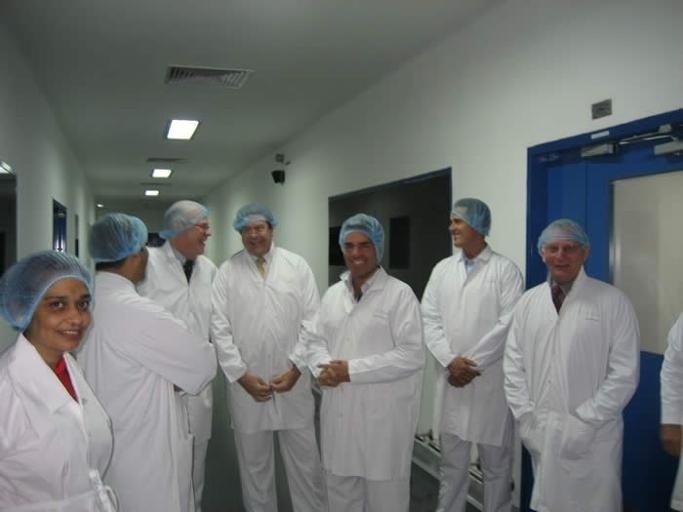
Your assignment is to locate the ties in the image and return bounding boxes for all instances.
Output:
[549,283,562,312]
[351,281,362,303]
[257,257,266,279]
[183,259,195,285]
[464,256,476,282]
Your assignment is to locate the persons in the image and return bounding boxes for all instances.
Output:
[74,211,219,512]
[502,219,641,512]
[211,203,323,512]
[299,213,421,512]
[659,311,682,512]
[0,250,119,512]
[421,197,526,512]
[132,199,217,512]
